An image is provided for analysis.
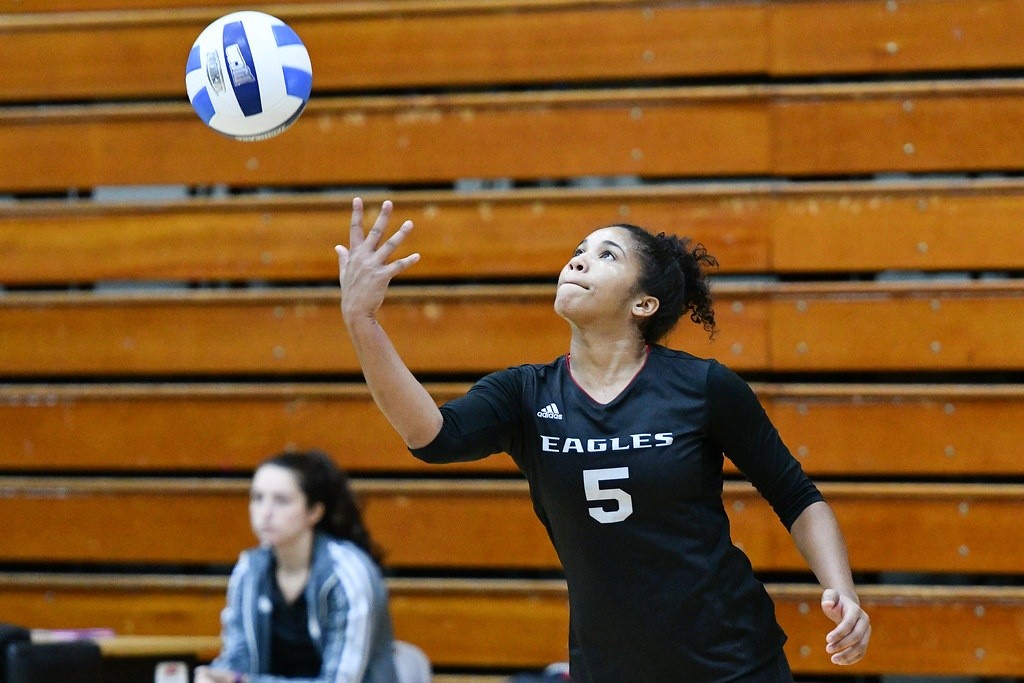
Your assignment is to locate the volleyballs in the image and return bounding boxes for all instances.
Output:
[184,10,313,144]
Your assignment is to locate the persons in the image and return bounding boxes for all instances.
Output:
[192,446,398,683]
[335,196,871,683]
